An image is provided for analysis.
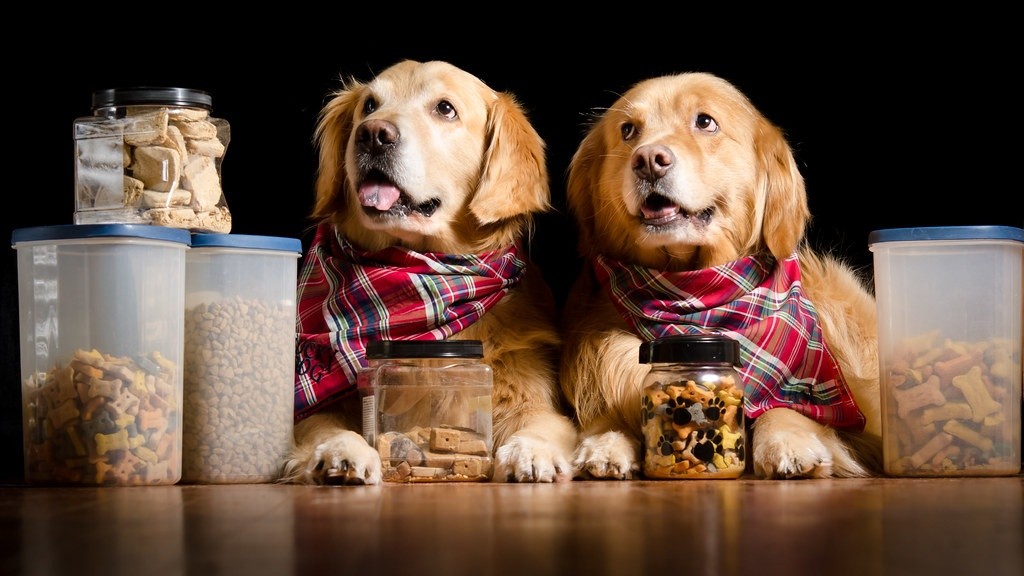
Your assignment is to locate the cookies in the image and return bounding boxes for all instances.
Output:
[375,424,493,479]
[883,330,1023,476]
[22,345,181,488]
[82,105,232,234]
[642,375,748,478]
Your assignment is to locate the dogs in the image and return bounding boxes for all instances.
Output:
[559,71,884,481]
[288,58,577,486]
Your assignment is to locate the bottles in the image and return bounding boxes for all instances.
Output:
[71,87,232,236]
[184,234,304,486]
[9,224,193,490]
[638,335,747,481]
[358,338,495,484]
[865,225,1024,478]
